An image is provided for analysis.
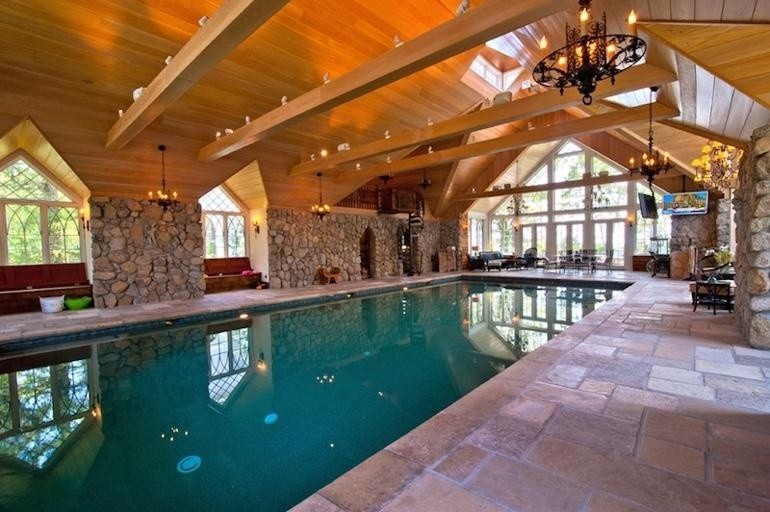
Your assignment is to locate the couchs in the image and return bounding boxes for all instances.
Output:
[479,251,502,266]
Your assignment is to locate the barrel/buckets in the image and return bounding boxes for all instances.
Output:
[39,292,65,314]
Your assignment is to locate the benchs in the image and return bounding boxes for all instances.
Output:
[204,257,261,293]
[0,263,92,314]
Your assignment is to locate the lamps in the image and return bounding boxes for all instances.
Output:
[148,145,182,211]
[530,1,647,108]
[628,86,744,191]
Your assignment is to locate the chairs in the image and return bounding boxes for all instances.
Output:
[516,248,537,269]
[542,248,614,274]
[466,253,486,272]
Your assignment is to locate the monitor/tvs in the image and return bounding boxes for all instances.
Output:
[639,194,658,218]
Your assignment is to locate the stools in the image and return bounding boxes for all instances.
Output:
[488,259,502,272]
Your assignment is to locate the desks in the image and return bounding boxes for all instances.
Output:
[497,258,524,271]
